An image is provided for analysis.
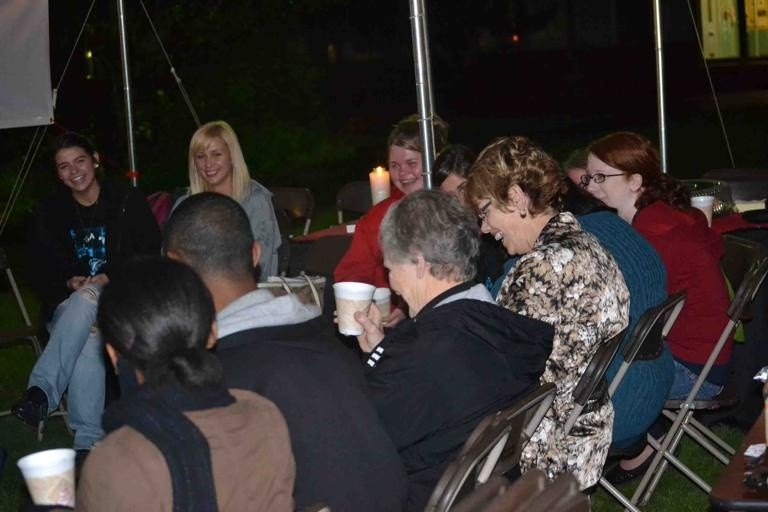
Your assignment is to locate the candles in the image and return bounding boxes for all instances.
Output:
[370,167,390,205]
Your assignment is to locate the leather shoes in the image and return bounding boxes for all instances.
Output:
[602,434,680,485]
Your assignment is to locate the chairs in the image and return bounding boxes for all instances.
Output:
[561,324,630,436]
[597,289,688,509]
[1,179,396,502]
[425,383,559,510]
[631,261,764,506]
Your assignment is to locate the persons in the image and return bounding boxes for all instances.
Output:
[169,120,282,284]
[11,132,156,469]
[51,256,295,512]
[332,113,746,511]
[161,193,408,512]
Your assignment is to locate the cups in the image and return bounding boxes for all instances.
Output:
[373,287,391,326]
[16,447,77,511]
[333,281,376,337]
[690,195,713,229]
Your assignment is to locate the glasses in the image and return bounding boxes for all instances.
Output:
[580,172,627,187]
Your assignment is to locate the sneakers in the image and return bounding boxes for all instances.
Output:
[11,387,49,428]
